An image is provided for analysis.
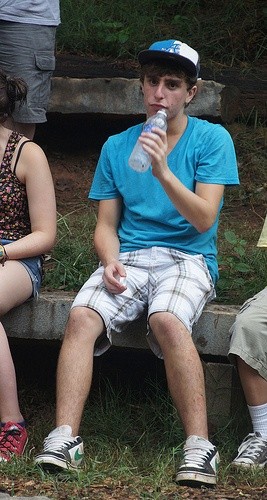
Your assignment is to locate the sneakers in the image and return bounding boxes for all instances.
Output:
[230,431,267,470]
[175,435,220,484]
[34,425,84,472]
[0,421,29,465]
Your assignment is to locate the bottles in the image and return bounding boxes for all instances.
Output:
[129,107,168,171]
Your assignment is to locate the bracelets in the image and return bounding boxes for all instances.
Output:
[0,245,8,265]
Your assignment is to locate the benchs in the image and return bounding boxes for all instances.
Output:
[47,77,243,128]
[1,292,243,432]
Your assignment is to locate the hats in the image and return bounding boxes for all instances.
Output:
[137,39,200,77]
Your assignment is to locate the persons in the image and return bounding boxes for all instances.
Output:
[227,218,267,472]
[33,40,240,484]
[0,0,61,141]
[0,70,56,465]
